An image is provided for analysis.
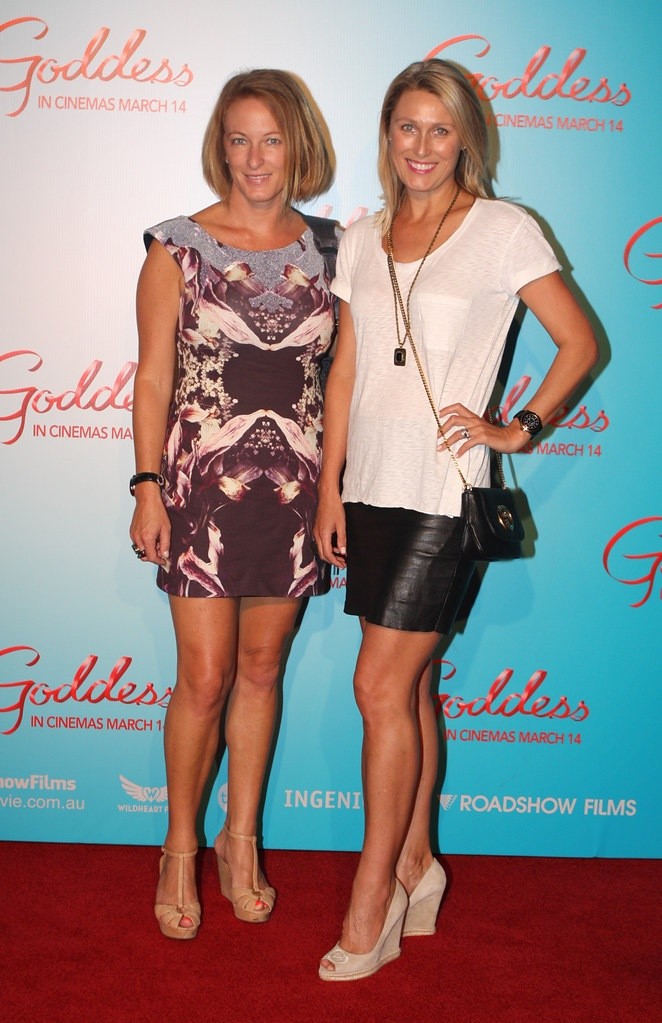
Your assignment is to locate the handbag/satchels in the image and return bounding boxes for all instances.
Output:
[460,488,525,563]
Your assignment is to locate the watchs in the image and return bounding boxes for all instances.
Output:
[129,472,161,496]
[513,410,543,440]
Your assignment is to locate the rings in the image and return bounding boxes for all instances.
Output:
[131,544,146,558]
[462,428,470,438]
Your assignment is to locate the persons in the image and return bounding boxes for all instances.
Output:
[314,59,600,981]
[128,69,347,938]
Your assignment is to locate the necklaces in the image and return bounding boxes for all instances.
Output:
[388,187,461,367]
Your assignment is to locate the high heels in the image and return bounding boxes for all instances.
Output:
[318,879,409,981]
[403,858,447,935]
[216,826,275,921]
[154,847,201,940]
